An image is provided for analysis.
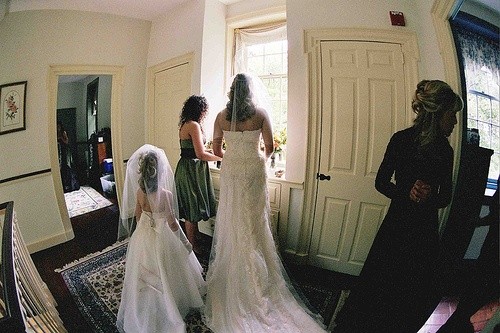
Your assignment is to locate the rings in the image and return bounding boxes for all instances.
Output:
[420,189,423,192]
[417,197,421,202]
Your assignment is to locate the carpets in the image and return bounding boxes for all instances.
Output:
[64,186,113,219]
[53,236,352,333]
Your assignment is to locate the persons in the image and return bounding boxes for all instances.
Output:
[170,94,224,258]
[120,144,208,308]
[471,172,499,300]
[57,119,74,169]
[328,78,461,332]
[213,73,274,275]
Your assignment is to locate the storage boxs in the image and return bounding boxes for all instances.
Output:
[103,158,113,173]
[99,174,116,194]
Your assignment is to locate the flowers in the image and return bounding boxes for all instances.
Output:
[206,137,226,169]
[273,129,289,154]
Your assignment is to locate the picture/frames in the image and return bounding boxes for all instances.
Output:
[0,81,28,135]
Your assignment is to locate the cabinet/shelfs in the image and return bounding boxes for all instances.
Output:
[93,143,110,183]
[439,148,495,264]
